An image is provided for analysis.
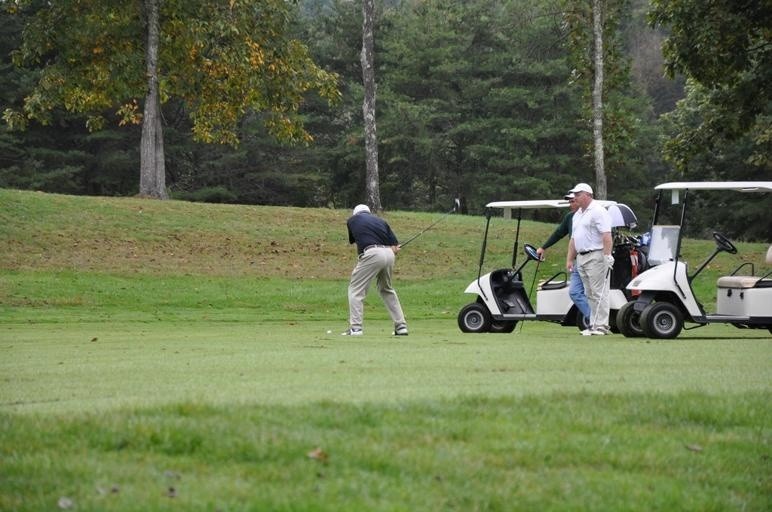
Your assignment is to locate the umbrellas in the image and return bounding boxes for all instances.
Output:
[602,203,638,228]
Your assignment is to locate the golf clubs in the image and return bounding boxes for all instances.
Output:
[515,253,541,335]
[396,198,460,248]
[582,266,610,336]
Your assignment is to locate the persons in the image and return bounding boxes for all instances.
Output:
[340,203,409,336]
[536,187,591,319]
[566,183,615,335]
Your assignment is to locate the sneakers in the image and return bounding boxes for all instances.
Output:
[580,325,612,335]
[342,328,364,335]
[393,328,409,334]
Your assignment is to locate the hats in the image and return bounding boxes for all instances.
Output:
[353,204,371,214]
[564,183,593,199]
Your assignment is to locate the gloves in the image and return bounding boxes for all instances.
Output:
[604,255,615,271]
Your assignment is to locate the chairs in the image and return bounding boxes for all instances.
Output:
[716,244,772,289]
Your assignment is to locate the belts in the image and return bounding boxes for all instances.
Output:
[579,251,593,254]
[365,245,392,249]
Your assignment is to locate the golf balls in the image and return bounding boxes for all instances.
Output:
[327,330,332,334]
